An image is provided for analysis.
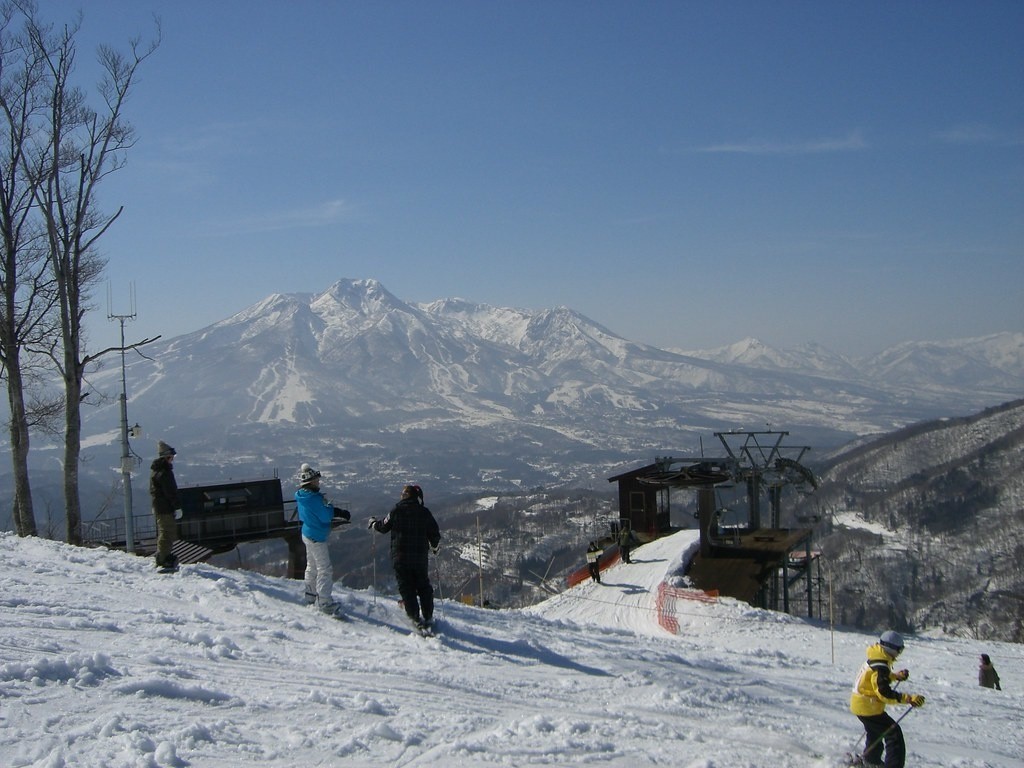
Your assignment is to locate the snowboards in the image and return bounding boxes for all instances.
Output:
[155,554,176,573]
[299,590,345,620]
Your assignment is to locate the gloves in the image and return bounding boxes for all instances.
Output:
[898,669,908,681]
[333,507,351,521]
[367,516,376,529]
[909,693,925,707]
[433,545,441,554]
[175,509,182,520]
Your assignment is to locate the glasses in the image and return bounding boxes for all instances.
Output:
[400,491,407,497]
[898,646,903,653]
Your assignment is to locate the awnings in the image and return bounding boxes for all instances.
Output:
[204,489,252,499]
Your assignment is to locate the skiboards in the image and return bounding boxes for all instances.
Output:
[396,598,434,638]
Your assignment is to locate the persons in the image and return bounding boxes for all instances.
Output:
[618,524,635,563]
[587,542,601,583]
[150,441,182,568]
[979,654,1002,690]
[484,597,489,608]
[850,631,925,768]
[368,486,441,628]
[295,464,341,613]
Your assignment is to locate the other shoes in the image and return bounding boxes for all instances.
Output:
[320,600,345,619]
[305,592,319,605]
[157,564,179,573]
[863,756,885,768]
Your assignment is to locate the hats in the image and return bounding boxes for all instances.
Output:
[880,630,904,655]
[300,463,321,485]
[159,441,176,456]
